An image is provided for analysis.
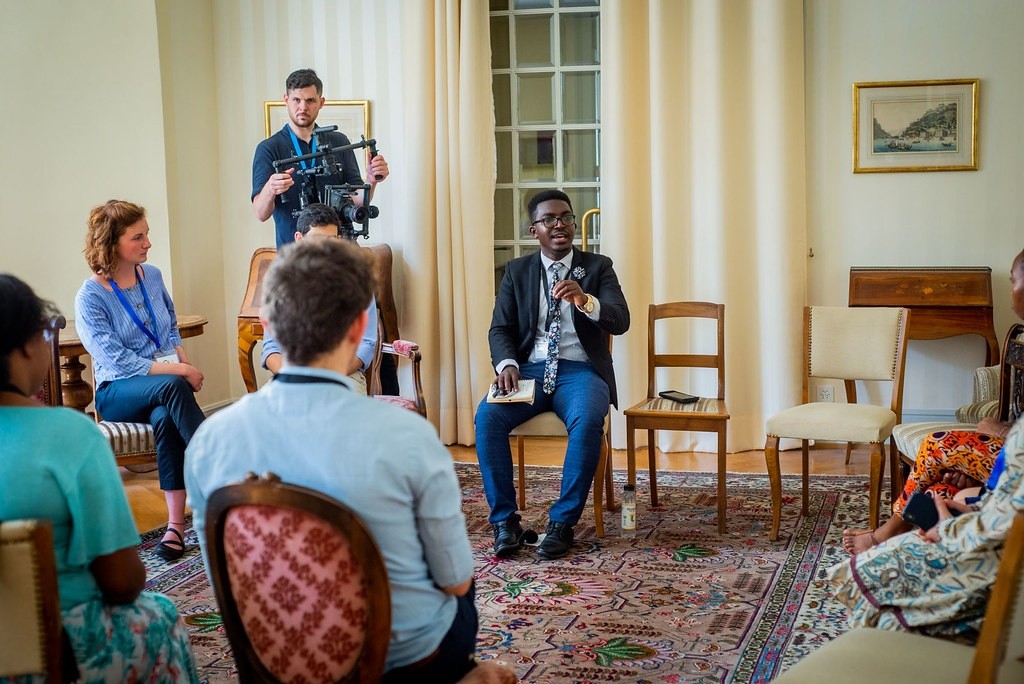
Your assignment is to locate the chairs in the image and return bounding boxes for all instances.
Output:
[765,306,911,542]
[368,308,426,418]
[892,323,1024,506]
[92,354,159,467]
[205,470,391,684]
[623,301,730,533]
[508,330,621,537]
[0,519,64,684]
[29,315,66,406]
[771,509,1024,684]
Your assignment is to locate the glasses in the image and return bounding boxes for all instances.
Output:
[532,215,576,228]
[38,326,55,341]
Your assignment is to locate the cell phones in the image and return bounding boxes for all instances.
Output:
[658,390,700,403]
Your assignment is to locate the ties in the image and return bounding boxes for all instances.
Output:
[543,260,564,395]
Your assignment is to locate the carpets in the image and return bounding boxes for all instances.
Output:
[139,460,892,683]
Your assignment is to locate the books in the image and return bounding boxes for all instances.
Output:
[486,378,536,406]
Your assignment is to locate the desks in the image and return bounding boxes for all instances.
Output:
[844,265,1000,466]
[59,316,210,417]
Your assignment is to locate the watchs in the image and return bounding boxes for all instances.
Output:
[576,294,595,313]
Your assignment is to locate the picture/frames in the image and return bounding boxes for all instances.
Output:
[265,101,371,183]
[852,79,980,174]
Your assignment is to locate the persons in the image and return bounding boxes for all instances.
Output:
[184,239,480,684]
[259,203,378,399]
[74,200,205,561]
[0,273,199,684]
[474,190,631,558]
[842,248,1024,557]
[826,415,1024,645]
[251,69,390,250]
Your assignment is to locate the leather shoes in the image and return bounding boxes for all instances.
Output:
[486,514,528,556]
[535,521,573,558]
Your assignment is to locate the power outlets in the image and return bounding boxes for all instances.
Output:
[816,386,834,402]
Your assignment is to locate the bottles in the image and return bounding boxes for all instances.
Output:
[620,484,636,540]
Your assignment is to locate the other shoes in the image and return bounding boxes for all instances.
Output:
[155,528,186,562]
[842,527,880,558]
